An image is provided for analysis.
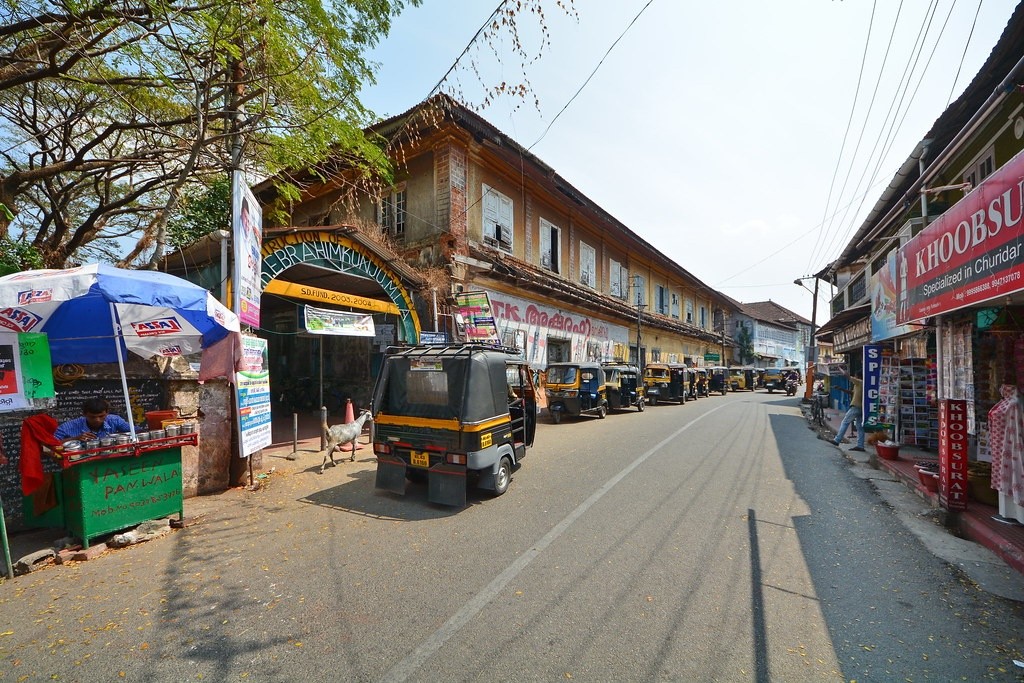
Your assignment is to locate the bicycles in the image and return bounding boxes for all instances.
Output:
[811,395,824,427]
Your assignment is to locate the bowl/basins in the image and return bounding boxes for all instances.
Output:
[875,444,901,460]
[933,474,939,495]
[913,464,937,486]
[918,469,938,493]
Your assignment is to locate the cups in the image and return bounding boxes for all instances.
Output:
[63,422,195,459]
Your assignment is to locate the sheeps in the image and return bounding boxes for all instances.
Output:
[320,408,373,474]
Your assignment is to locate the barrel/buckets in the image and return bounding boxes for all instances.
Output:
[145,410,178,430]
[161,419,185,429]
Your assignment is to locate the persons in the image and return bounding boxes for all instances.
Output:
[827,364,866,452]
[54,398,142,442]
[507,381,518,400]
[785,370,799,392]
[240,196,250,237]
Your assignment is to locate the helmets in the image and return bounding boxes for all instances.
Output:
[791,370,795,373]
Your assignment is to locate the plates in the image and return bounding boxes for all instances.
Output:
[871,282,886,321]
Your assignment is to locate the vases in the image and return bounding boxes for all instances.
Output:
[914,462,998,507]
[873,443,900,459]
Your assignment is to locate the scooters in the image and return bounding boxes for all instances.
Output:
[784,376,798,396]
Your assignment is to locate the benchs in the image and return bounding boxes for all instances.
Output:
[580,377,598,394]
[621,378,637,395]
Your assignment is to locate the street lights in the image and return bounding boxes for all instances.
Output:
[793,280,817,403]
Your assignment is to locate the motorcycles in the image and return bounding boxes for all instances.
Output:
[369,341,537,508]
[544,361,802,424]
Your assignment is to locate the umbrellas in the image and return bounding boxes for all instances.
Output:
[0,263,240,443]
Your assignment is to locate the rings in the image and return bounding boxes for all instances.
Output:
[85,438,87,440]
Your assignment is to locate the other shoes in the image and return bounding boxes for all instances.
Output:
[848,446,865,452]
[827,439,840,446]
